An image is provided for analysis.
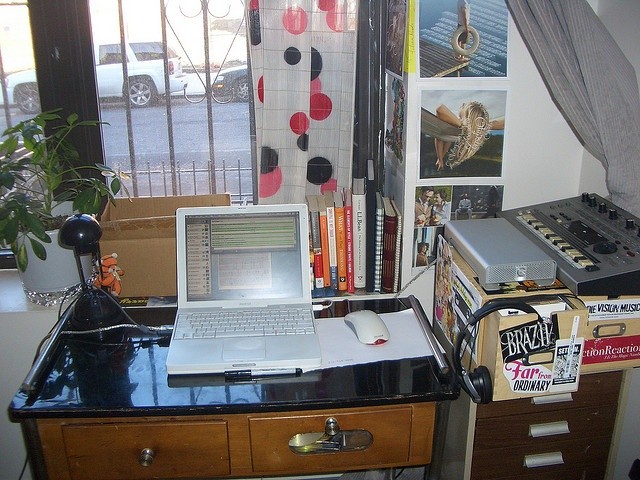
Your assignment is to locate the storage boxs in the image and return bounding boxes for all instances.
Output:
[98,192,231,298]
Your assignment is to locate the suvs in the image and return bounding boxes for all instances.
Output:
[4,38,188,115]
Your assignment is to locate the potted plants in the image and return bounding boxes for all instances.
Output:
[0,108,135,307]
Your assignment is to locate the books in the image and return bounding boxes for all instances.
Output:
[306,160,402,292]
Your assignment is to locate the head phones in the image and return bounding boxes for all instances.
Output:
[452,299,544,405]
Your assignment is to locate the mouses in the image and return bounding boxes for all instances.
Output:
[342,310,389,347]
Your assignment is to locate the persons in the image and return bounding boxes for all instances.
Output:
[432,191,452,224]
[416,186,434,226]
[416,242,429,267]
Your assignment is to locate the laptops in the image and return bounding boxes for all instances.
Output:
[161,201,323,377]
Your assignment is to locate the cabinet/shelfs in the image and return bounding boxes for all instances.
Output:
[433,235,634,480]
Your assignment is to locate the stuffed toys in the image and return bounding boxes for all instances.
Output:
[94,252,125,297]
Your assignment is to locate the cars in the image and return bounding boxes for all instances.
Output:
[212,65,249,103]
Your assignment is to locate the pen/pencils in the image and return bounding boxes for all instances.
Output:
[224,368,303,379]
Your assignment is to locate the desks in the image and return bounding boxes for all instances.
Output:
[7,296,461,479]
